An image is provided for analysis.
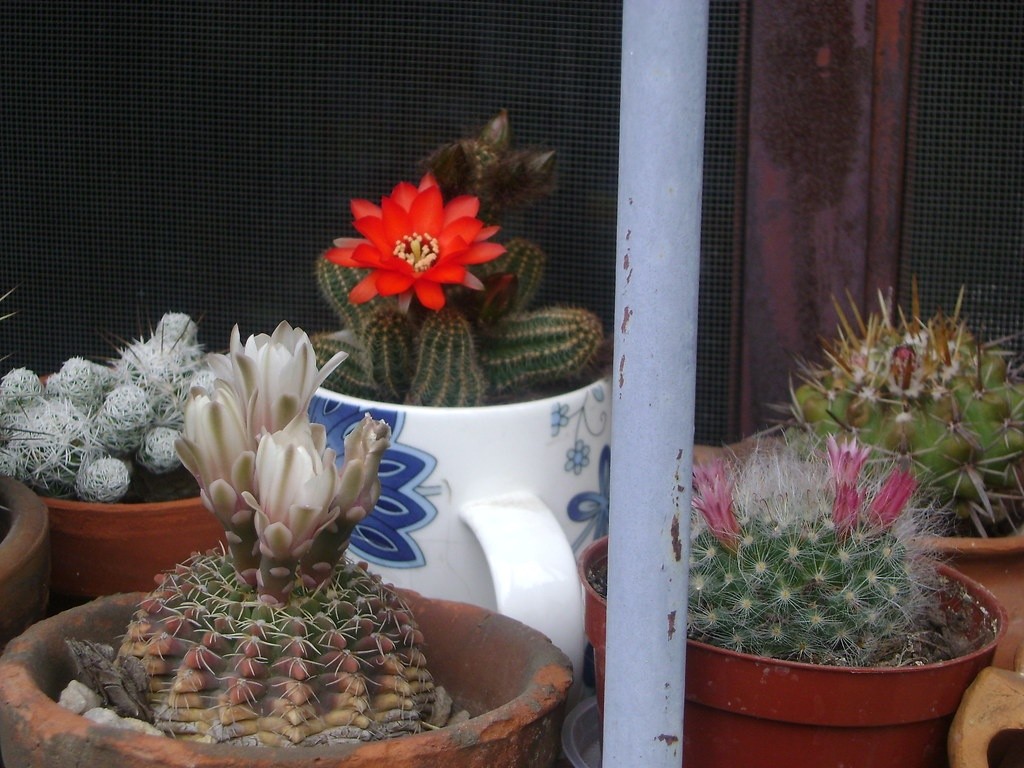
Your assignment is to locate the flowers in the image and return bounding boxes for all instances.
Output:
[100,306,449,747]
[0,313,227,509]
[681,423,943,660]
[310,114,602,395]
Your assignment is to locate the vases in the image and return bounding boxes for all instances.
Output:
[574,528,1007,768]
[311,351,611,691]
[0,473,53,644]
[41,492,228,601]
[0,572,581,768]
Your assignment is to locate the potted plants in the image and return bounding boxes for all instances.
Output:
[755,277,1024,669]
